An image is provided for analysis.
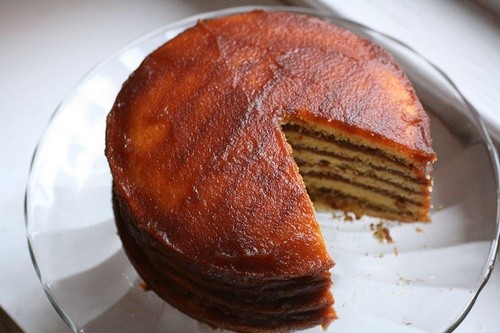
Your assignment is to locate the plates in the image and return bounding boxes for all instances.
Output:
[24,5,500,333]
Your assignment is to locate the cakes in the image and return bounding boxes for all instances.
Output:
[104,9,436,333]
